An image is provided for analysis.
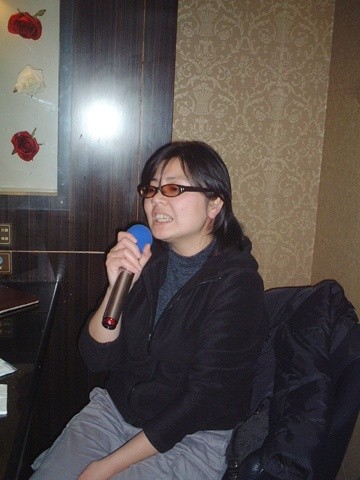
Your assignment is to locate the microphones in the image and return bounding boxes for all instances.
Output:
[102,224,153,329]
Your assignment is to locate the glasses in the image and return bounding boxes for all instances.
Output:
[137,183,213,198]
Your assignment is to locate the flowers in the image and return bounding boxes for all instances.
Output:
[7,7,48,41]
[10,65,47,98]
[10,128,45,163]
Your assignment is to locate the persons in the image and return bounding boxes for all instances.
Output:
[31,141,265,480]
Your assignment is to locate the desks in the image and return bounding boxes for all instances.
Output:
[0,281,61,480]
